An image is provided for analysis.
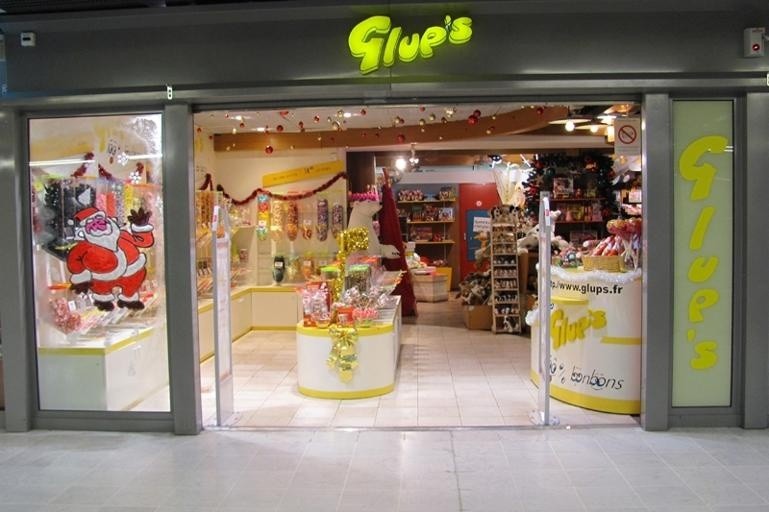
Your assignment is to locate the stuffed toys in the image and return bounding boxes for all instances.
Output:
[348,198,400,259]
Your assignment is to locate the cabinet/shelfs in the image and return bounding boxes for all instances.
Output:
[549,174,608,257]
[491,220,522,334]
[397,196,454,268]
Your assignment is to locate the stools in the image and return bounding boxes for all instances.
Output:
[411,268,452,302]
